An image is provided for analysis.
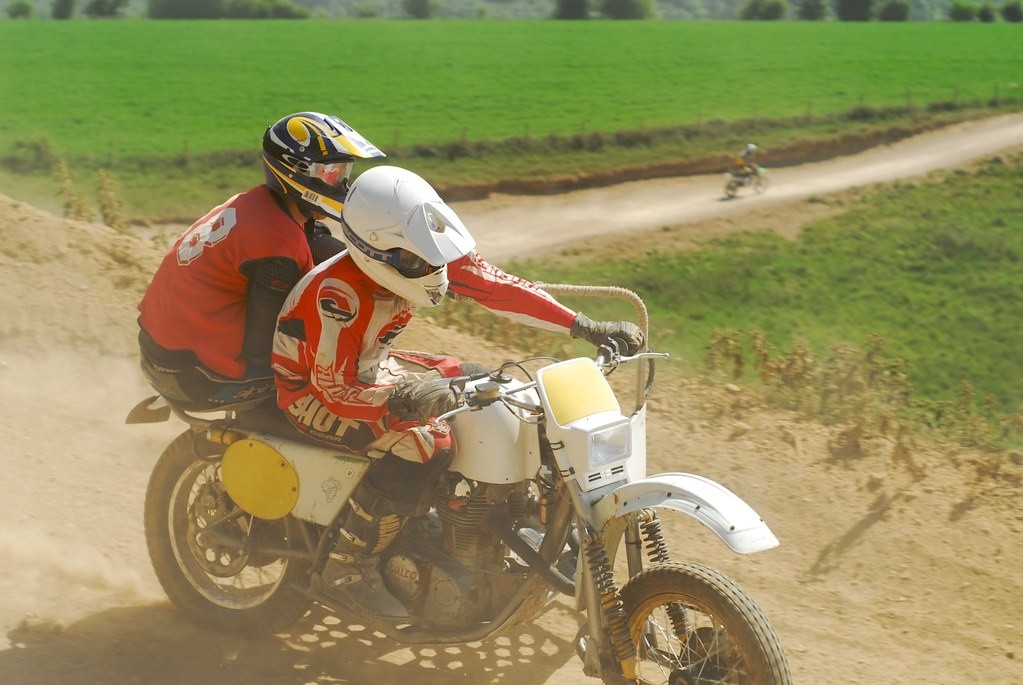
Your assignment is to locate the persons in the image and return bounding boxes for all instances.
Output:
[730,144,759,186]
[271,165,647,624]
[137,112,386,413]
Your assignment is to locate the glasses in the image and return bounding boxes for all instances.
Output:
[386,247,446,279]
[308,161,355,187]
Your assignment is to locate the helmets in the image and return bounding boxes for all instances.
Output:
[261,112,387,222]
[340,166,478,308]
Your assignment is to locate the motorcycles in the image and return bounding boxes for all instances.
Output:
[725,165,768,197]
[127,334,792,685]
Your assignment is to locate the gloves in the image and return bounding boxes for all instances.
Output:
[387,379,466,426]
[569,310,645,357]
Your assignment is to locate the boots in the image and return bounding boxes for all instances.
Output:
[316,483,412,624]
